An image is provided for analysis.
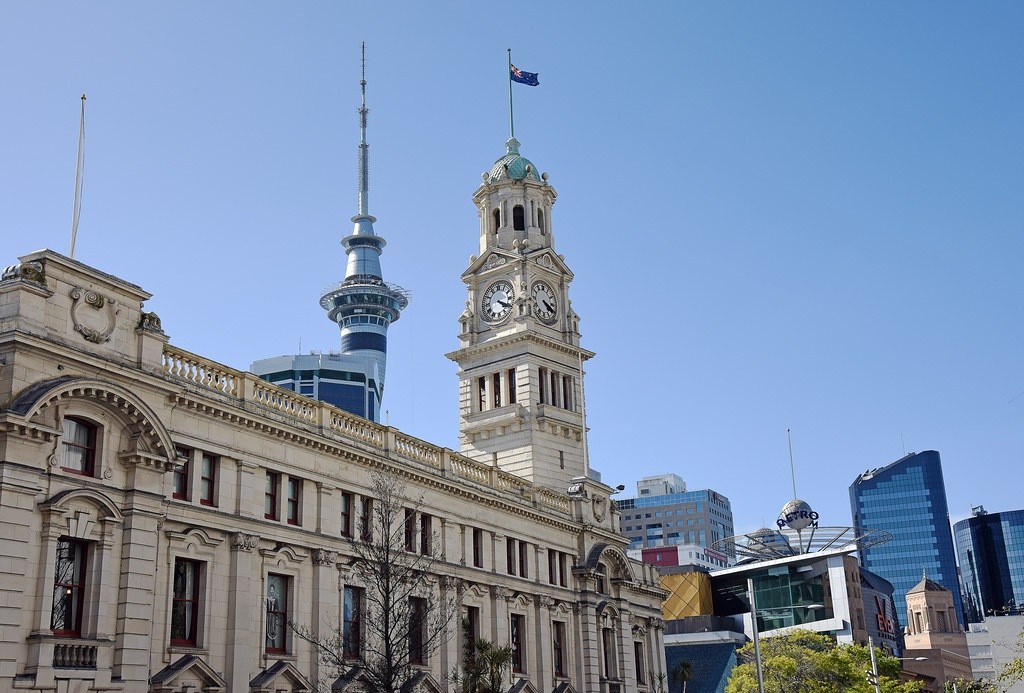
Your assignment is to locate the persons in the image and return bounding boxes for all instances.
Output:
[267,585,279,648]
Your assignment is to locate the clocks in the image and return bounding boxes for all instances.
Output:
[531,280,557,323]
[482,280,515,322]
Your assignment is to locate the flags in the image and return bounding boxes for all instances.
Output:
[508,63,540,86]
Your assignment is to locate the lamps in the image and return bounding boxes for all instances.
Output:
[613,485,625,494]
